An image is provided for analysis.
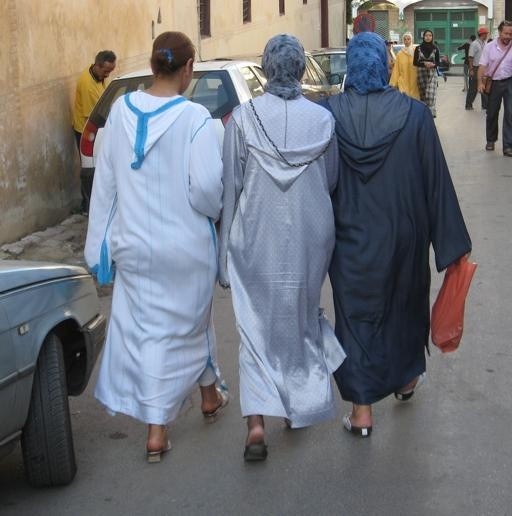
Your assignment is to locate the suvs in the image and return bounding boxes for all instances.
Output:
[78,60,269,207]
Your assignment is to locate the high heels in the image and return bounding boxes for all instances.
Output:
[146,441,171,465]
[202,387,230,424]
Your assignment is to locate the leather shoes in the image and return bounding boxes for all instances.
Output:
[504,148,512,156]
[486,141,494,150]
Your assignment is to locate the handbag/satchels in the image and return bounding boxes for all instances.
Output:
[482,76,492,94]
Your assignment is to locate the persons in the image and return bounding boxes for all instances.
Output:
[388,30,420,101]
[457,34,476,93]
[464,28,488,110]
[67,49,118,218]
[487,38,493,43]
[83,32,225,459]
[413,29,441,119]
[382,38,396,56]
[477,20,512,158]
[315,29,475,436]
[216,36,341,465]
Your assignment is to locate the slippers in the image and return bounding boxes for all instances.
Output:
[395,372,428,401]
[244,442,267,461]
[343,410,373,438]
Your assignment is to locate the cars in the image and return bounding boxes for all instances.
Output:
[308,46,349,85]
[393,44,421,59]
[438,54,450,71]
[0,259,107,490]
[216,49,344,106]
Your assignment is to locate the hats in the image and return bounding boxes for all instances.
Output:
[478,28,490,35]
